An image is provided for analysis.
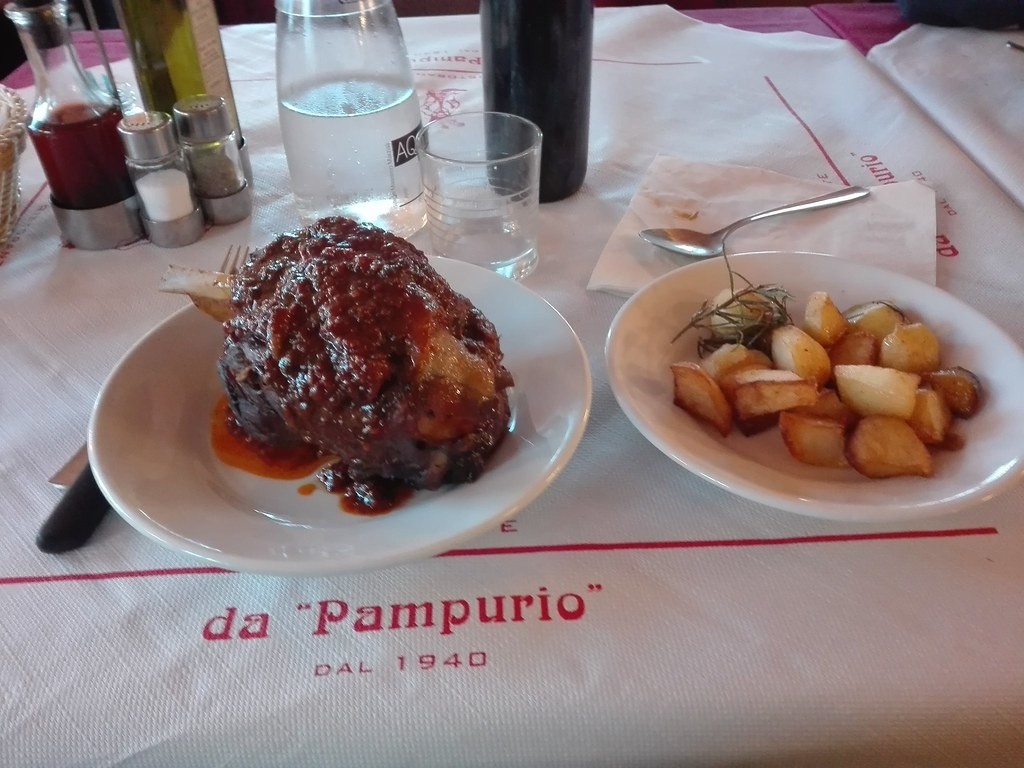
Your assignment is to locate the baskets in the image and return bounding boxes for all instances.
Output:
[0,82,32,265]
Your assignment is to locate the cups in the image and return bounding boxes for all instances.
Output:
[415,110,542,281]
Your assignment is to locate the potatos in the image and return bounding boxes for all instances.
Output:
[670,289,980,477]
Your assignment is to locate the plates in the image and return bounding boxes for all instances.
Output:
[605,249,1024,523]
[86,255,591,574]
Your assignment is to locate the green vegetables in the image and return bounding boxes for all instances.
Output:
[670,243,796,358]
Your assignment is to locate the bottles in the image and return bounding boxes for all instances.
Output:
[173,94,243,200]
[478,0,593,203]
[117,110,197,223]
[5,0,133,208]
[271,0,425,240]
[113,0,242,147]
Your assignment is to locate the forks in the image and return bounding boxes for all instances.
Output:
[46,244,258,484]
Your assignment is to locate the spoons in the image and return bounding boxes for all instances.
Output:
[637,185,871,256]
